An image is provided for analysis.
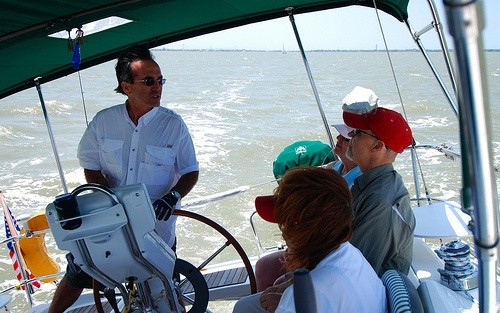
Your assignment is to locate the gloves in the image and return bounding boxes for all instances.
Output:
[152,192,178,221]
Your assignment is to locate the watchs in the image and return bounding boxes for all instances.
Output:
[170,191,181,203]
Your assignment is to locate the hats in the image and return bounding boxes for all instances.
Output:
[255,195,280,223]
[331,122,357,140]
[343,107,413,154]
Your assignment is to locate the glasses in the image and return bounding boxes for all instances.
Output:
[353,128,389,150]
[133,76,166,86]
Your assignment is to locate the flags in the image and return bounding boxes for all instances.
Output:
[1,190,40,294]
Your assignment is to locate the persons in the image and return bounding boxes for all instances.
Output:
[232,107,416,313]
[255,123,358,294]
[47,46,199,313]
[273,167,388,313]
[255,194,338,313]
